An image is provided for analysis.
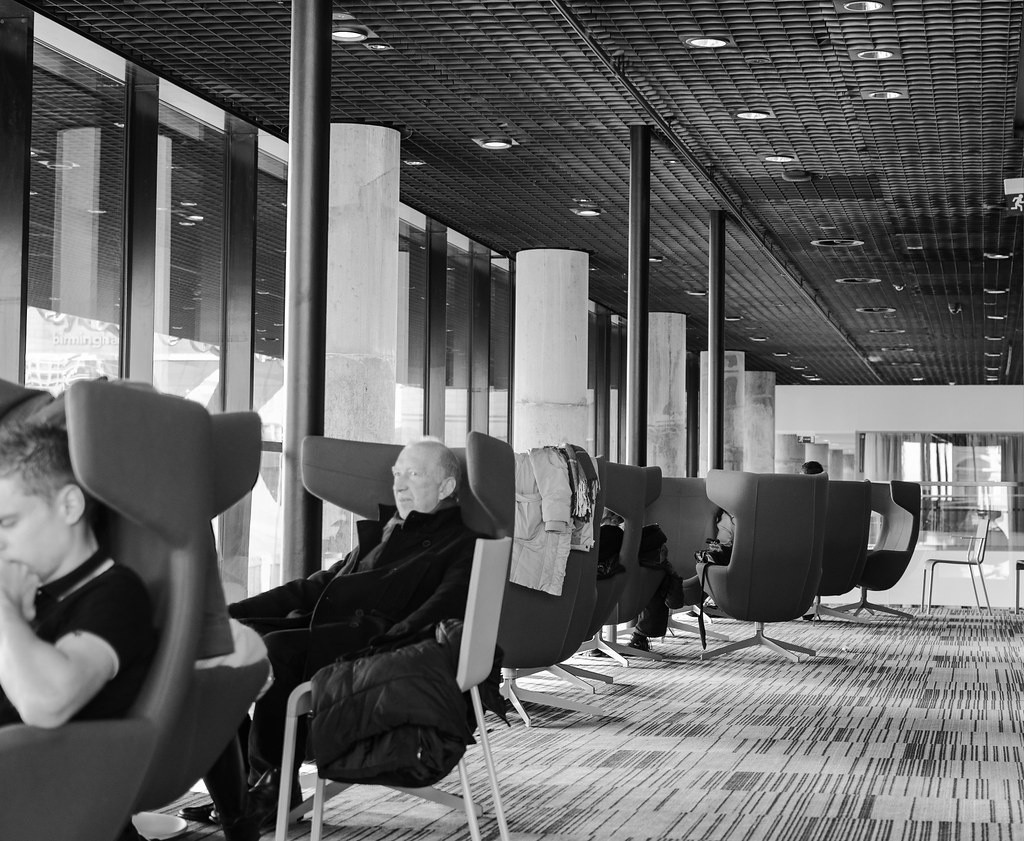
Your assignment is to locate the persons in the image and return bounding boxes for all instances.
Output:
[800,461,823,475]
[0,420,153,730]
[682,508,735,588]
[590,507,672,657]
[177,438,488,825]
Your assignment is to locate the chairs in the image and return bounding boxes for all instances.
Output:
[301,433,516,583]
[0,377,273,841]
[921,518,996,615]
[574,461,664,668]
[491,442,604,727]
[832,479,920,620]
[810,478,873,626]
[616,476,730,642]
[275,535,512,841]
[695,468,829,663]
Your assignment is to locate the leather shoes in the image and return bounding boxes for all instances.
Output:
[180,767,304,825]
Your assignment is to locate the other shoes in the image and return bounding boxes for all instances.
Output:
[626,633,649,652]
